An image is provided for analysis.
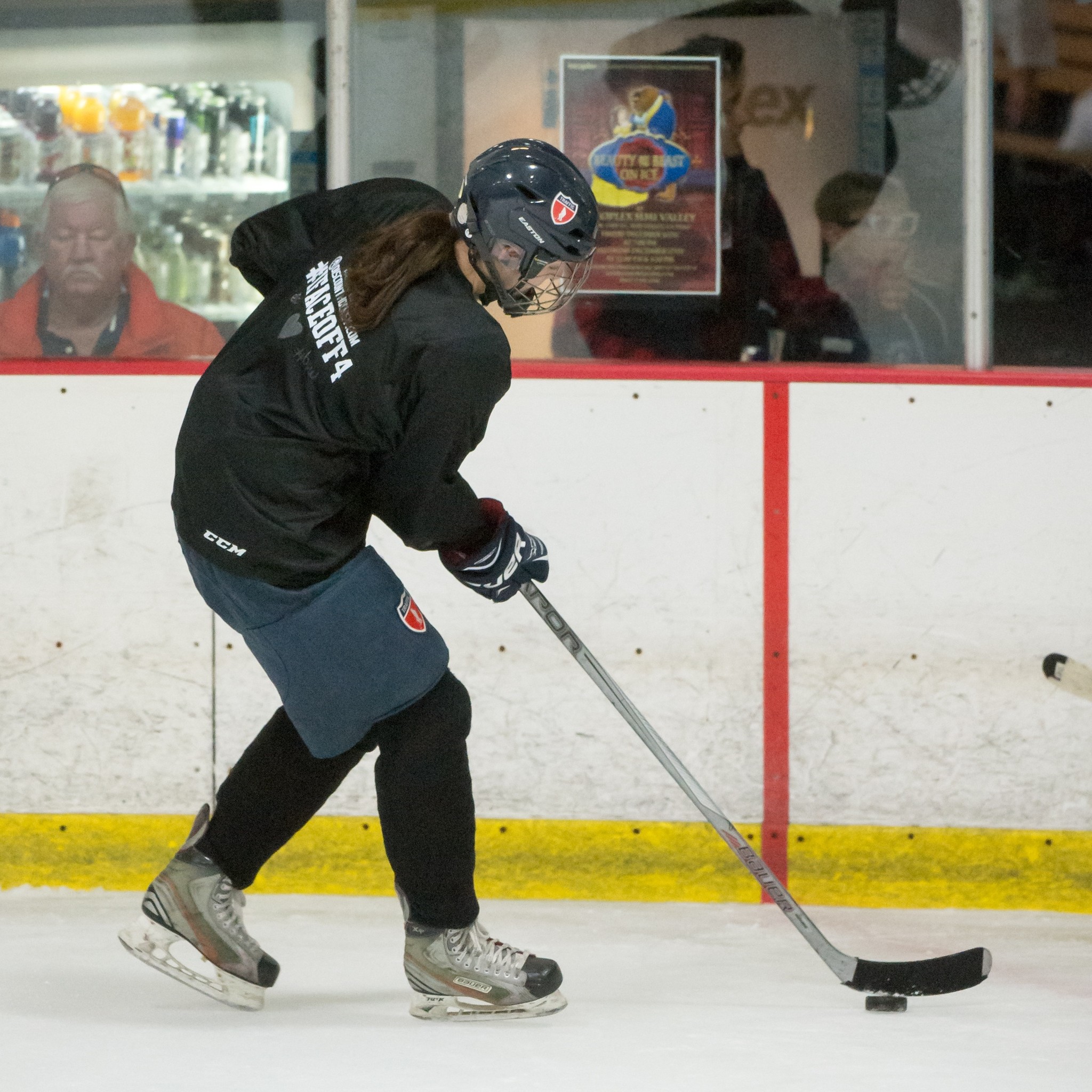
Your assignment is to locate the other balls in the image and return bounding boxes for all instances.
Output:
[863,997,910,1015]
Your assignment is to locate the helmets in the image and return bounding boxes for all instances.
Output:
[467,139,600,318]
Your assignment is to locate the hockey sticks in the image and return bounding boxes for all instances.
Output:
[1041,652,1092,700]
[520,575,993,996]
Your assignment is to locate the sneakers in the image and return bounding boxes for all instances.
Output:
[396,881,568,1020]
[118,804,279,1010]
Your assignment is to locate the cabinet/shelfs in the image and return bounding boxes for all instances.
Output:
[0,121,293,326]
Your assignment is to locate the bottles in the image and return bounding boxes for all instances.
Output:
[0,82,293,316]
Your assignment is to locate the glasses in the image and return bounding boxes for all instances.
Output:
[842,210,923,240]
[47,163,129,206]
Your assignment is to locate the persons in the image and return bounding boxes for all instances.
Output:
[565,35,869,360]
[0,162,225,358]
[812,170,967,365]
[117,139,599,1021]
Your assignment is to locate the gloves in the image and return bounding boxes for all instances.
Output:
[438,497,549,602]
